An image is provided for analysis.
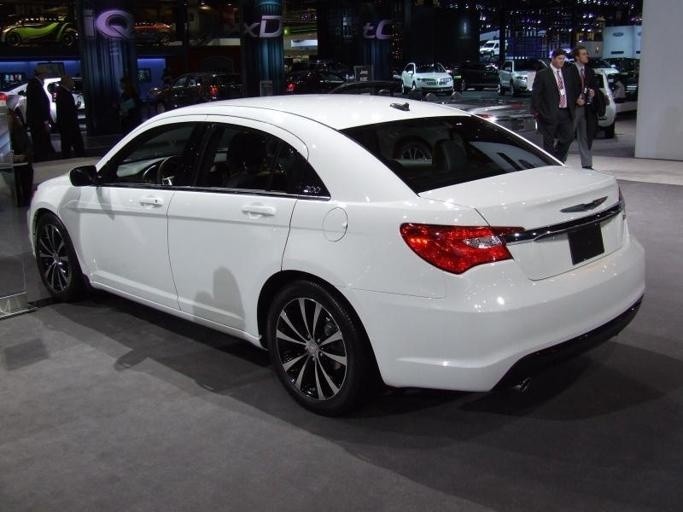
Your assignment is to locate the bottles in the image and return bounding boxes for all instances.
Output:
[583,86,588,101]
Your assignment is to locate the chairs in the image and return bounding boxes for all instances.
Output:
[224,132,282,192]
[430,139,452,175]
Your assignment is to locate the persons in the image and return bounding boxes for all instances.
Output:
[27,64,85,161]
[118,76,143,134]
[529,44,596,171]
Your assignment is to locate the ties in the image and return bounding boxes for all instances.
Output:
[556,70,566,108]
[580,68,585,96]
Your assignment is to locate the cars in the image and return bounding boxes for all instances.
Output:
[479,39,507,57]
[1,7,80,52]
